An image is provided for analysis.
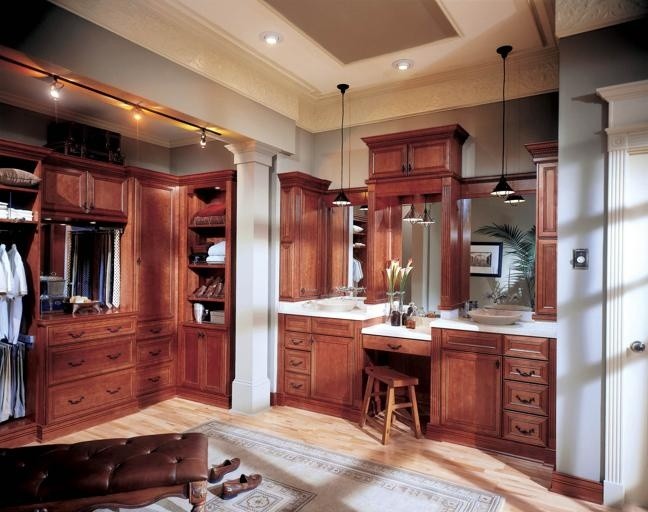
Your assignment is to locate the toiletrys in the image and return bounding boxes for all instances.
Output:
[473,301,480,308]
[463,300,473,318]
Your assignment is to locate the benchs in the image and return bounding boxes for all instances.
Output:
[1,429,216,512]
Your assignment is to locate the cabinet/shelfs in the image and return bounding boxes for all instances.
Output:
[175,170,238,409]
[272,313,383,423]
[33,147,143,445]
[117,166,180,410]
[277,182,330,303]
[358,121,464,180]
[0,131,45,452]
[425,327,560,472]
[529,154,558,324]
[362,334,435,357]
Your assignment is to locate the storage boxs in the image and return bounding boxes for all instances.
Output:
[209,310,224,326]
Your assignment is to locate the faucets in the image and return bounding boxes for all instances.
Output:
[356,286,366,297]
[335,286,356,297]
[508,294,520,303]
[492,295,508,304]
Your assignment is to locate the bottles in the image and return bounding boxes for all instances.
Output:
[474,301,480,308]
[407,303,435,318]
[391,307,416,329]
[463,301,474,318]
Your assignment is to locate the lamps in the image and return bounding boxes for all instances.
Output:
[501,193,525,206]
[402,192,423,225]
[489,44,515,196]
[391,57,415,75]
[357,205,369,211]
[258,31,281,45]
[418,200,436,228]
[0,53,220,149]
[331,83,355,209]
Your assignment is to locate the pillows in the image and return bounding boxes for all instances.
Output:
[352,224,364,232]
[0,165,43,187]
[0,169,42,186]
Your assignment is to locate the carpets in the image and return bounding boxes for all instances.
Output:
[81,418,509,512]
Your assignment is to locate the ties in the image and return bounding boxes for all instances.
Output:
[63,224,122,308]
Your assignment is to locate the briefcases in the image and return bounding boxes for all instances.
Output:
[44,122,125,164]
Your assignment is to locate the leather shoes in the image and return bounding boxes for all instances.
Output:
[222,474,262,498]
[210,457,240,482]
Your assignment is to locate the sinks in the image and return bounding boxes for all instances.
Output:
[305,299,361,314]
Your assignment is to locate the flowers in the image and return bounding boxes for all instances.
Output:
[397,256,414,292]
[380,258,401,295]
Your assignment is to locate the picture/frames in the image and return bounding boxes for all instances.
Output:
[468,240,505,279]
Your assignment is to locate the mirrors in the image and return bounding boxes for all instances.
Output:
[460,190,537,313]
[398,202,444,318]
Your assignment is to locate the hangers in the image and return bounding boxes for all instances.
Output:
[351,251,364,264]
[1,229,17,251]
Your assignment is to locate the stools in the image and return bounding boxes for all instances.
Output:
[360,362,424,446]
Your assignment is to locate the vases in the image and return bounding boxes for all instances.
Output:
[384,291,398,323]
[397,290,406,315]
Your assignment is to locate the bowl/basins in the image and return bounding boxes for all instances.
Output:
[315,299,357,312]
